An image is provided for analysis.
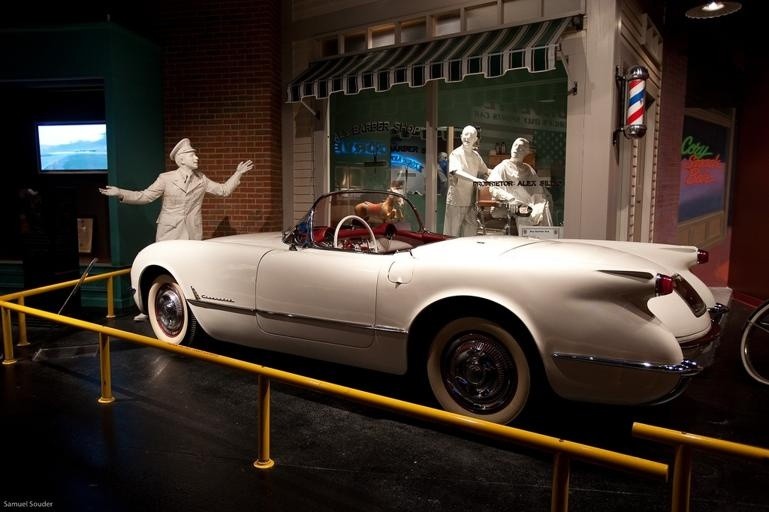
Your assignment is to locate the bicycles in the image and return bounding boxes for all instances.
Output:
[738,299,769,389]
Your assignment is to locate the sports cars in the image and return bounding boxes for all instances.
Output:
[130,187,730,430]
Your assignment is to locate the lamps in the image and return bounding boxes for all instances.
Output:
[685,0,742,20]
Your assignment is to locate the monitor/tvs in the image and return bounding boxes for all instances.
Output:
[33,120,109,174]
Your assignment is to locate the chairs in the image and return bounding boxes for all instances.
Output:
[474,184,510,236]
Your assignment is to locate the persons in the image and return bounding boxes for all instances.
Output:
[442,124,494,237]
[98,137,255,323]
[485,137,554,239]
[435,151,449,196]
